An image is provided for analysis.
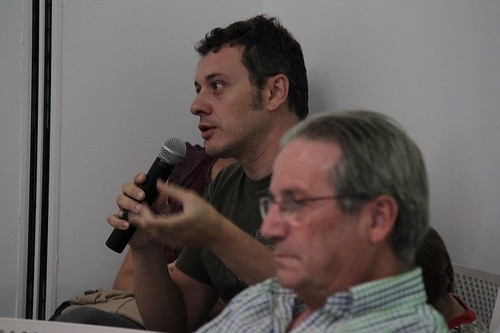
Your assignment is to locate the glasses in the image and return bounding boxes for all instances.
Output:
[259,196,350,219]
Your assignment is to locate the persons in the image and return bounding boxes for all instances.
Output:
[54,11,310,333]
[195,107,448,333]
[414,226,488,333]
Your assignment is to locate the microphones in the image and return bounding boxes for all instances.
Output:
[105,138,186,254]
